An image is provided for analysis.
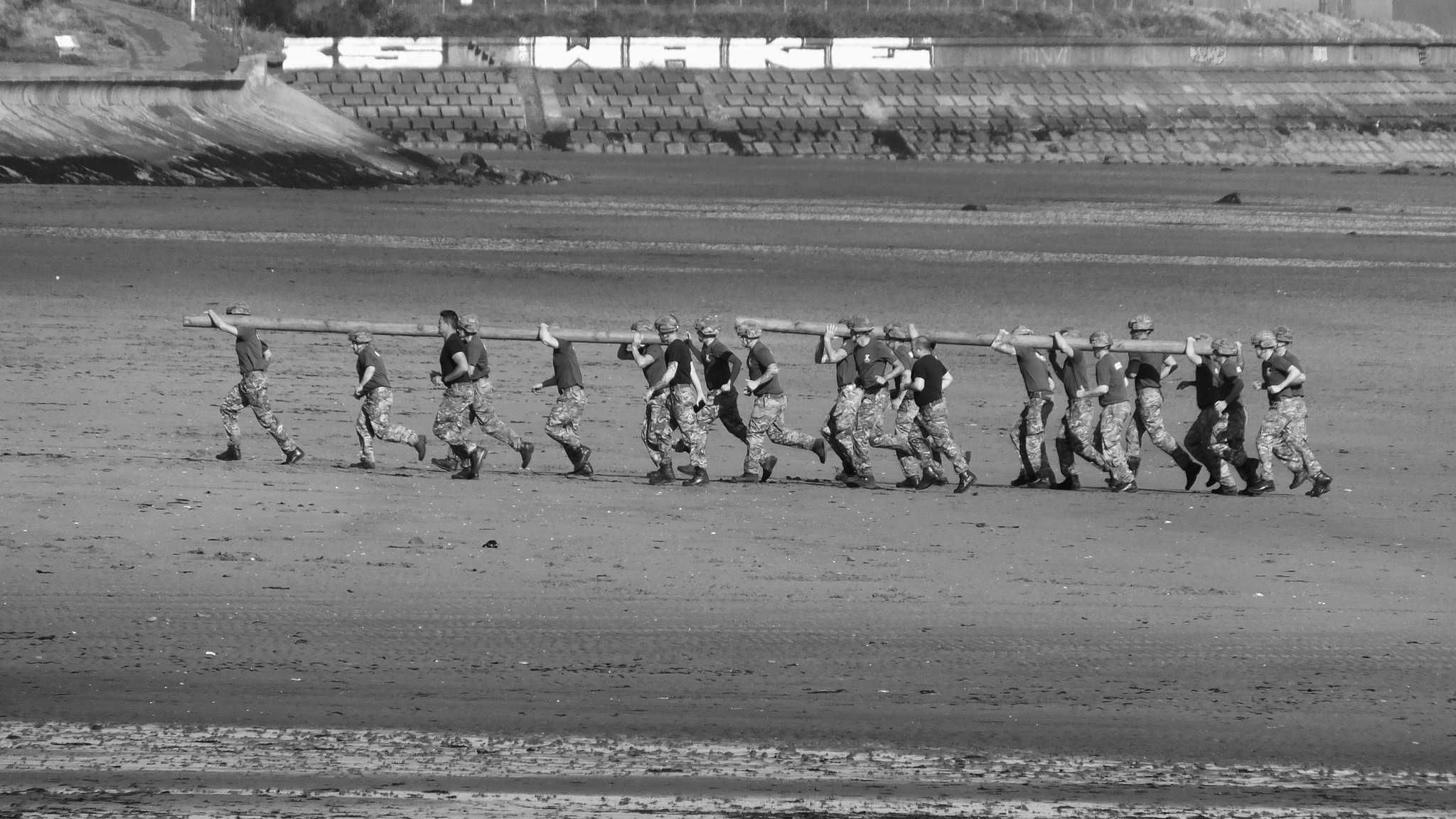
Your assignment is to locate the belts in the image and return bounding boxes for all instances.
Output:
[559,385,581,392]
[1275,395,1292,401]
[670,383,691,391]
[653,387,669,396]
[919,398,943,412]
[450,382,472,388]
[365,386,391,396]
[1068,396,1090,403]
[837,382,855,393]
[1029,391,1053,401]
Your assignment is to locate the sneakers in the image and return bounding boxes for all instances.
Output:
[350,461,375,470]
[414,434,426,460]
[644,436,977,495]
[1010,458,1335,499]
[430,438,597,482]
[280,446,305,466]
[215,445,241,460]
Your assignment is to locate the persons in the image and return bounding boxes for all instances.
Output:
[1202,338,1263,495]
[642,314,708,486]
[992,325,1057,488]
[678,316,778,480]
[841,315,917,487]
[882,322,972,487]
[900,336,977,493]
[1077,330,1144,492]
[1048,327,1111,490]
[1248,326,1333,497]
[815,317,860,484]
[1105,313,1202,490]
[732,319,827,482]
[1176,334,1237,495]
[203,301,676,484]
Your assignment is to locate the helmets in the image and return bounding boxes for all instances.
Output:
[839,314,908,340]
[457,313,480,332]
[736,320,764,338]
[347,325,373,343]
[537,319,562,329]
[631,315,722,336]
[226,301,251,315]
[1010,312,1294,356]
